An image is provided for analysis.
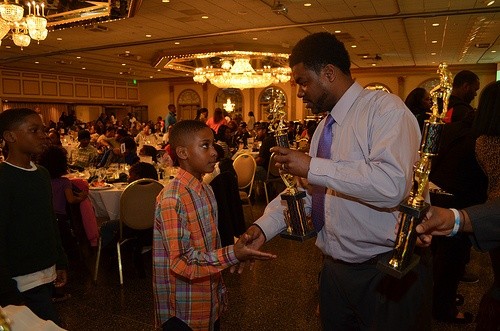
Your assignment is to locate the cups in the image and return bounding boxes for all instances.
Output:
[61,133,177,182]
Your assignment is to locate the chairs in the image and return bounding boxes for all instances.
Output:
[65,178,110,289]
[253,151,282,205]
[117,177,166,288]
[230,153,257,222]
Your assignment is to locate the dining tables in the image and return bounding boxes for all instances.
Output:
[87,182,125,221]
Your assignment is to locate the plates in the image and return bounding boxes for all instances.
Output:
[88,183,113,190]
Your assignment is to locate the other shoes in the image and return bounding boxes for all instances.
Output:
[454,311,475,325]
[459,274,480,284]
[455,295,465,305]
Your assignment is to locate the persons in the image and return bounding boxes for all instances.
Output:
[404,70,500,323]
[229,32,437,330]
[1,104,322,266]
[152,119,277,331]
[0,108,68,326]
[394,198,499,251]
[267,89,288,131]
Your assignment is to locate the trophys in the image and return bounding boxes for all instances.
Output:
[375,60,453,279]
[267,101,317,240]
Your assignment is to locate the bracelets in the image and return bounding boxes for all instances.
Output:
[445,208,461,237]
[457,209,465,236]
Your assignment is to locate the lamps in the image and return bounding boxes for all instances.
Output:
[0,0,144,52]
[155,44,293,90]
